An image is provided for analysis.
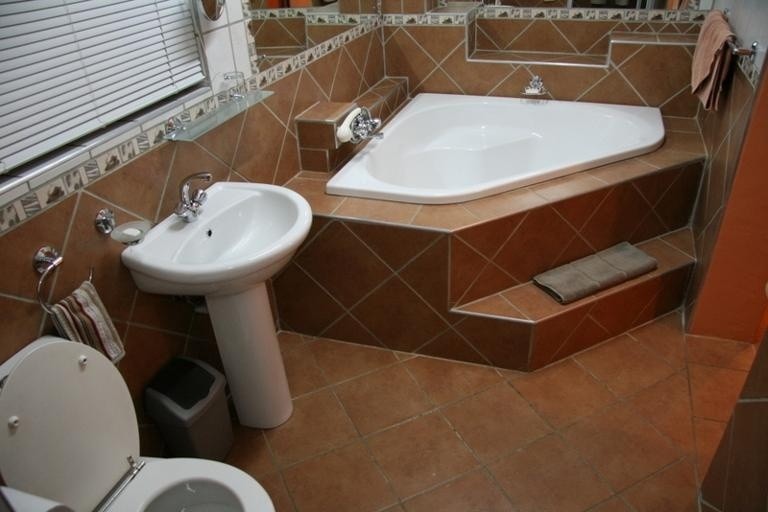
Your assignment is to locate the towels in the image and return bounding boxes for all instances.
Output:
[53,280,126,363]
[690,12,736,111]
[532,241,657,303]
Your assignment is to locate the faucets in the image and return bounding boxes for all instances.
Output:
[174,172,213,222]
[347,106,385,145]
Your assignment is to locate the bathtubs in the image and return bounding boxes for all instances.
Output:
[327,92,667,204]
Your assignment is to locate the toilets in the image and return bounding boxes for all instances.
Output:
[0,335,276,511]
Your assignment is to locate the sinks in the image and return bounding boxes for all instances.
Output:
[120,181,313,296]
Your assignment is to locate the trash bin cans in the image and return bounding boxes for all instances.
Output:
[144,355,235,463]
